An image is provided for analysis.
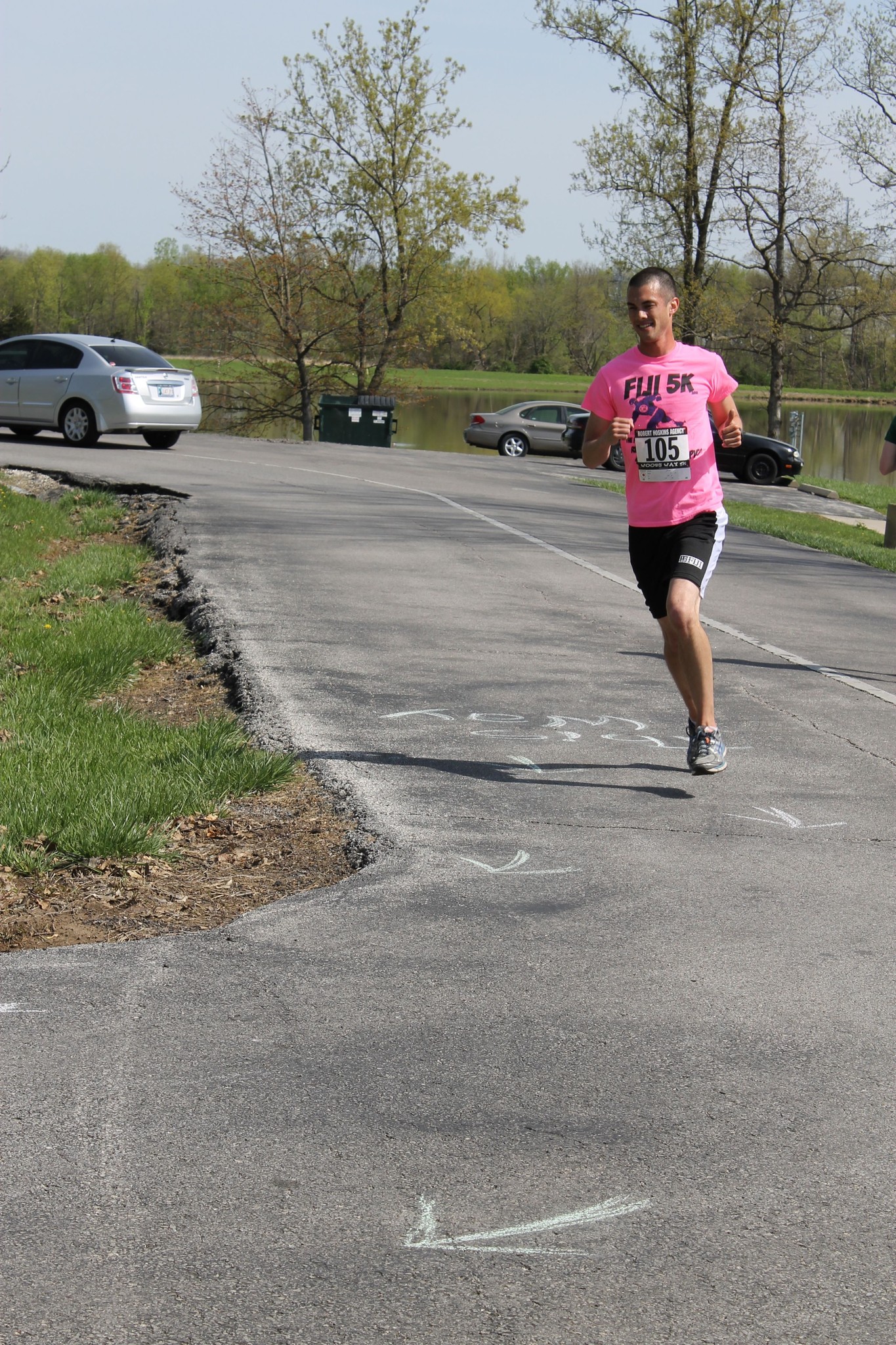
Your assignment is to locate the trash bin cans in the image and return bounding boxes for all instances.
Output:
[314,393,399,449]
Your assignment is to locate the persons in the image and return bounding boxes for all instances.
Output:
[879,416,896,476]
[581,267,742,776]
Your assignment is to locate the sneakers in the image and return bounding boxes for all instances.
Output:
[692,727,724,771]
[685,719,727,776]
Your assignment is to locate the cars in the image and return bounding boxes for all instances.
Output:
[561,408,805,485]
[0,330,202,449]
[464,401,592,457]
